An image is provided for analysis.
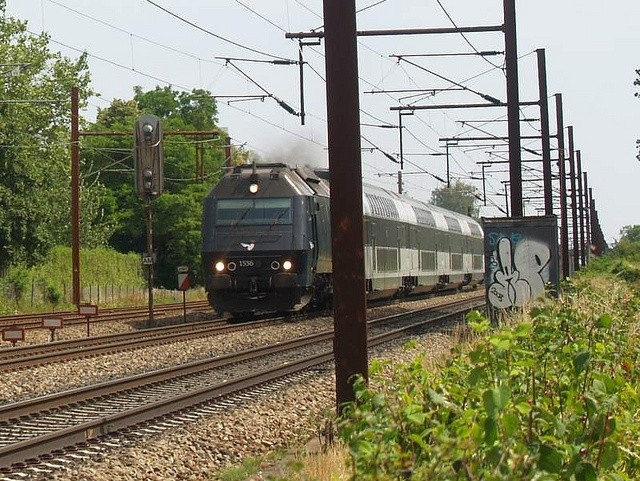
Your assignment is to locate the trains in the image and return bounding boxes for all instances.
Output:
[201,160,485,323]
